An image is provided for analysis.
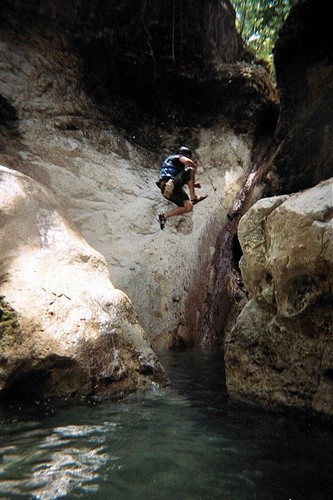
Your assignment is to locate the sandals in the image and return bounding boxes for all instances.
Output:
[191,195,208,205]
[158,213,166,231]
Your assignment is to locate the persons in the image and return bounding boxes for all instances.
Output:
[155,146,209,229]
